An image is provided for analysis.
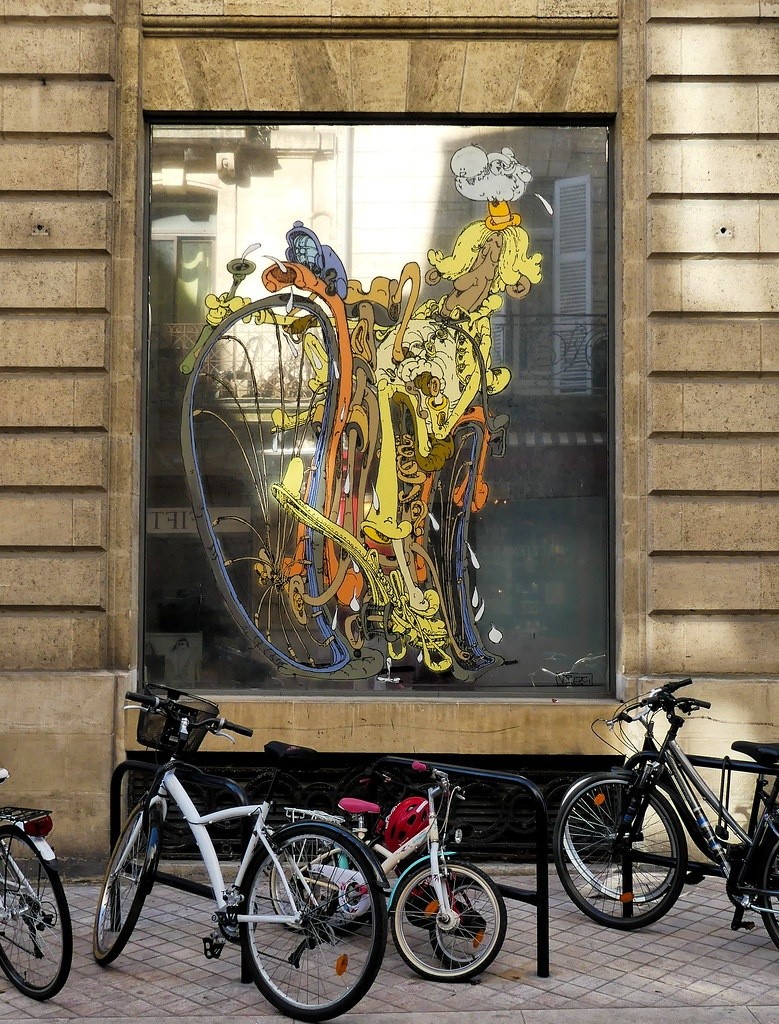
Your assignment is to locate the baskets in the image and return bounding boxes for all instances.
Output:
[136,683,219,758]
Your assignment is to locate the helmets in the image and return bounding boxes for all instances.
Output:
[384,797,429,863]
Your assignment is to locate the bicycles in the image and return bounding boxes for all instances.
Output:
[91,682,388,1023]
[0,765,74,1001]
[270,760,508,983]
[552,678,779,950]
